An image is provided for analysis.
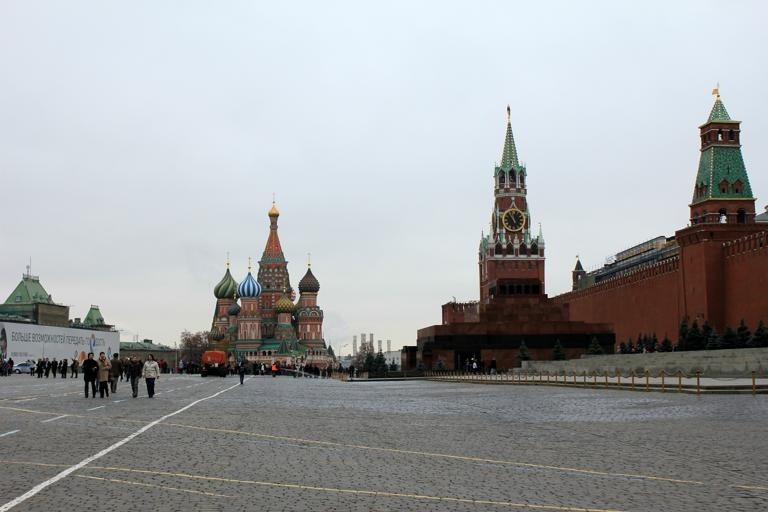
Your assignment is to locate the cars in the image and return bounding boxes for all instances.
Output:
[12,363,38,374]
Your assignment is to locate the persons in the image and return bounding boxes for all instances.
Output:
[0,357,14,377]
[161,360,168,373]
[29,358,79,378]
[82,351,161,397]
[438,359,443,370]
[462,355,505,375]
[239,363,245,384]
[178,360,355,379]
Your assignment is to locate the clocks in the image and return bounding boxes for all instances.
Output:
[501,208,526,233]
